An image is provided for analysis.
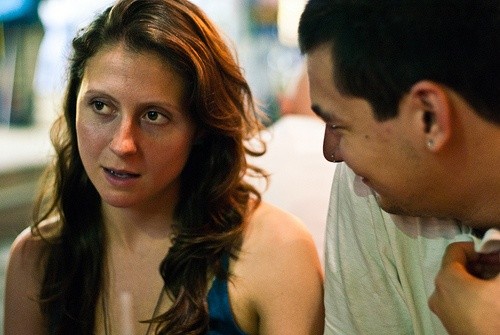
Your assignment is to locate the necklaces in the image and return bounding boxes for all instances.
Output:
[98,229,171,334]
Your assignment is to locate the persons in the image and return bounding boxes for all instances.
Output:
[0,0,325,334]
[249,59,339,278]
[298,0,499,334]
